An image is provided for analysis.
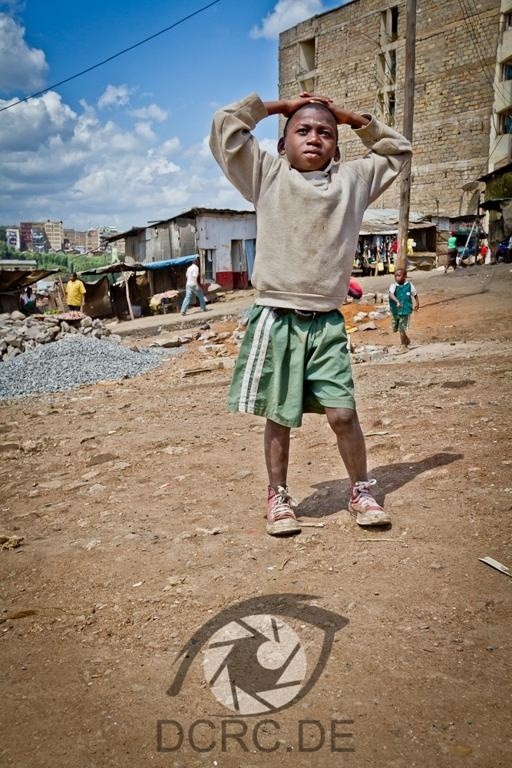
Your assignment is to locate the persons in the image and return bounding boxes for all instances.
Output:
[342,277,362,305]
[388,267,420,355]
[209,91,413,536]
[180,258,209,316]
[16,285,41,320]
[65,272,86,312]
[444,230,457,272]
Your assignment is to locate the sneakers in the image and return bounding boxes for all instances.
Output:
[348,479,391,526]
[266,485,301,534]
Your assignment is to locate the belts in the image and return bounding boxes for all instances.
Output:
[274,308,320,320]
